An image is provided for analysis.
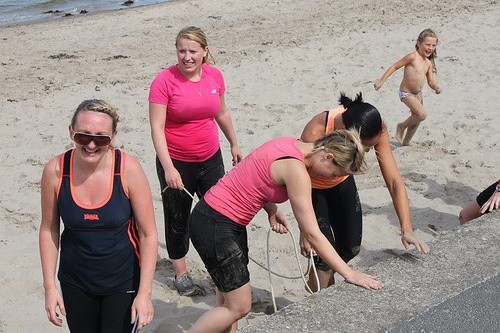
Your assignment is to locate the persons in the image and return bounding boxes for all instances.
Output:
[188,129,386,333]
[39,99,158,333]
[301,92,430,294]
[459,179,500,225]
[148,26,244,296]
[373,29,441,146]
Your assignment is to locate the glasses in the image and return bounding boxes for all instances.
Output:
[70,126,114,146]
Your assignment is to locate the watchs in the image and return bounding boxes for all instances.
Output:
[496,184,500,192]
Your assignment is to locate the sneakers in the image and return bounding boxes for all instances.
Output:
[174,272,199,296]
